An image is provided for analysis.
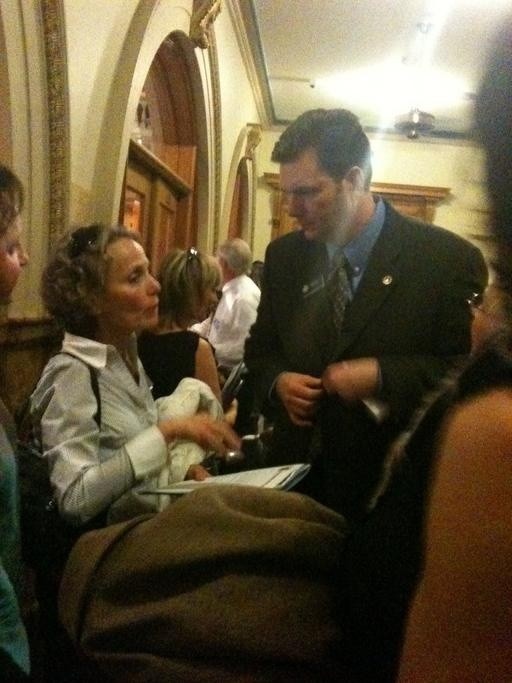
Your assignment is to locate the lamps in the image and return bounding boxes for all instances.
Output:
[394,104,435,139]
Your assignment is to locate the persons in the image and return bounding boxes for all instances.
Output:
[18,224,241,683]
[0,166,33,683]
[396,25,512,683]
[188,238,261,380]
[138,248,239,427]
[245,110,488,519]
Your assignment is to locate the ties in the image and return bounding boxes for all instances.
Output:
[319,254,353,358]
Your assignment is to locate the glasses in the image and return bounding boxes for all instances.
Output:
[68,225,98,260]
[185,247,197,275]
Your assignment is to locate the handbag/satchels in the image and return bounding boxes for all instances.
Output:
[14,437,71,578]
